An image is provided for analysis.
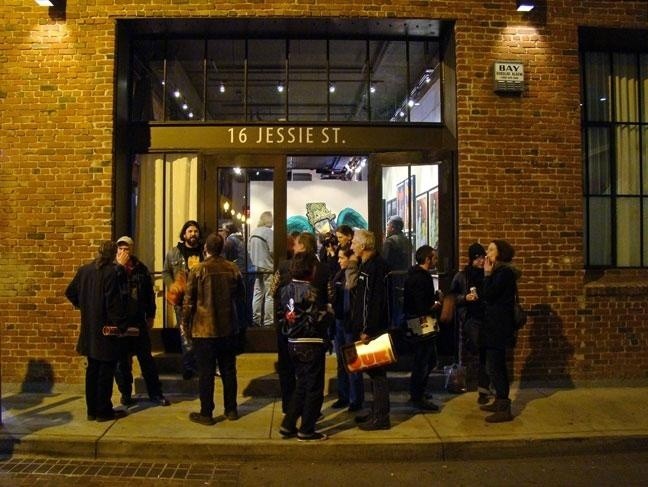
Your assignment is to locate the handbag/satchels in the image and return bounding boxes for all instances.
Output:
[440,295,456,322]
[400,276,440,343]
[461,316,480,354]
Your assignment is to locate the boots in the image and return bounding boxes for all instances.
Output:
[485,399,512,422]
[480,397,497,412]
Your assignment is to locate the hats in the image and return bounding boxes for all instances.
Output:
[468,243,486,261]
[117,236,134,248]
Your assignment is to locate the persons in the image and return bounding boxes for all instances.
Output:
[64,240,128,423]
[350,229,395,431]
[116,235,170,406]
[402,244,454,413]
[479,239,523,424]
[180,233,247,426]
[319,223,354,276]
[247,211,275,328]
[330,248,365,412]
[273,232,337,421]
[161,220,206,379]
[221,221,257,329]
[382,215,414,324]
[450,242,492,405]
[278,251,335,442]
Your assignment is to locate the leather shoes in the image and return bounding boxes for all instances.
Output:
[87,414,96,420]
[97,410,128,422]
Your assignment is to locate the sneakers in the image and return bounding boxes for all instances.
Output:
[120,398,132,404]
[190,412,214,425]
[150,396,171,406]
[413,401,439,411]
[425,392,432,398]
[331,400,349,410]
[478,391,490,405]
[348,406,363,413]
[224,409,238,421]
[297,432,327,442]
[277,427,298,438]
[354,416,370,424]
[359,419,390,431]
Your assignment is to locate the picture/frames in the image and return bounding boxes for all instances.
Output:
[395,175,415,234]
[387,198,397,224]
[406,235,416,267]
[414,192,428,255]
[427,185,439,279]
